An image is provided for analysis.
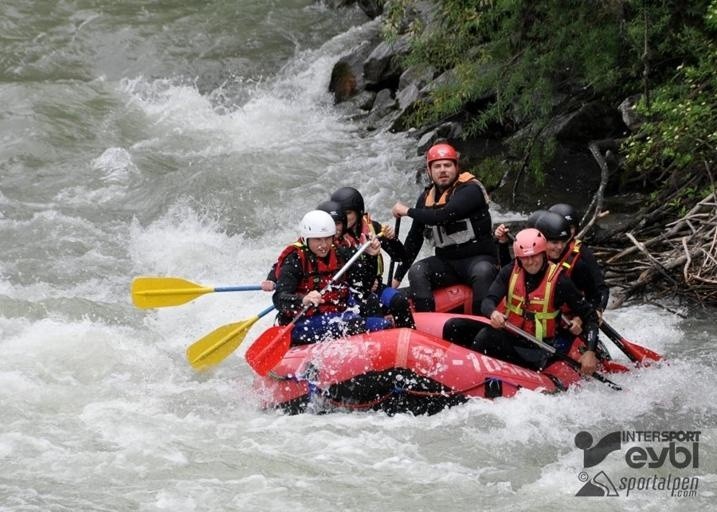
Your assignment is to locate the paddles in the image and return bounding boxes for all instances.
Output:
[244,242,375,375]
[562,312,631,373]
[602,320,662,366]
[131,278,279,308]
[188,303,274,375]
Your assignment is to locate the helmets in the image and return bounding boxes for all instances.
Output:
[330,188,364,209]
[316,201,347,236]
[513,203,578,266]
[427,144,459,178]
[299,210,336,246]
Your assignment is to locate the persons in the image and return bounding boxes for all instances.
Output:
[259,188,416,347]
[439,203,612,377]
[389,143,501,319]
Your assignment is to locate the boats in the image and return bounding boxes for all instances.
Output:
[252,312,604,418]
